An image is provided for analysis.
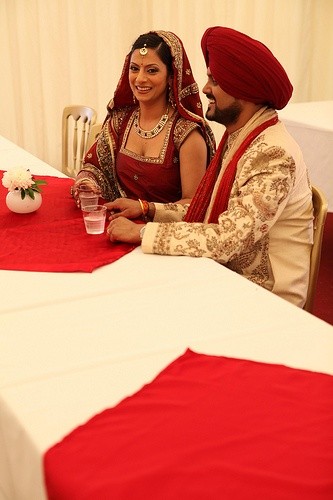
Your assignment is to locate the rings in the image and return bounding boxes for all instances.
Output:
[107,229,110,234]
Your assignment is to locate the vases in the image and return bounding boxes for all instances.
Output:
[5,190,43,215]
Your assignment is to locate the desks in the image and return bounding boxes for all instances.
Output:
[275,98,333,216]
[0,132,333,500]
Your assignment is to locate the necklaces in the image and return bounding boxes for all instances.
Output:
[134,105,169,139]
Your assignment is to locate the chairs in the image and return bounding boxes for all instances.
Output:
[61,102,99,174]
[303,183,325,310]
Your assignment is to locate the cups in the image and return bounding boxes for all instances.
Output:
[79,184,99,211]
[82,204,107,235]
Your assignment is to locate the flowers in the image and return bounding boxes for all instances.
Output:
[2,164,48,200]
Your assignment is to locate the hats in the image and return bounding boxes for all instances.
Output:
[200,25,294,110]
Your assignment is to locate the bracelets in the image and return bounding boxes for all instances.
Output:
[138,199,148,216]
[70,178,95,195]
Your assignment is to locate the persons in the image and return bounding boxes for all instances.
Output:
[71,31,217,208]
[103,25,314,308]
